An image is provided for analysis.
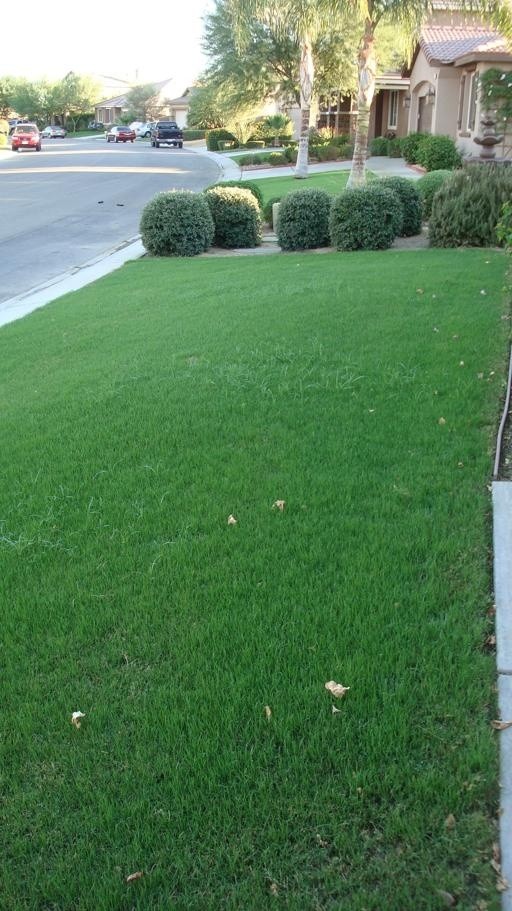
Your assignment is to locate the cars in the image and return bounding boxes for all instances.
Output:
[9,120,66,152]
[87,121,103,129]
[106,119,183,149]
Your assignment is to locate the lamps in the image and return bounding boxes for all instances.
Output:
[425,88,434,105]
[403,95,410,108]
